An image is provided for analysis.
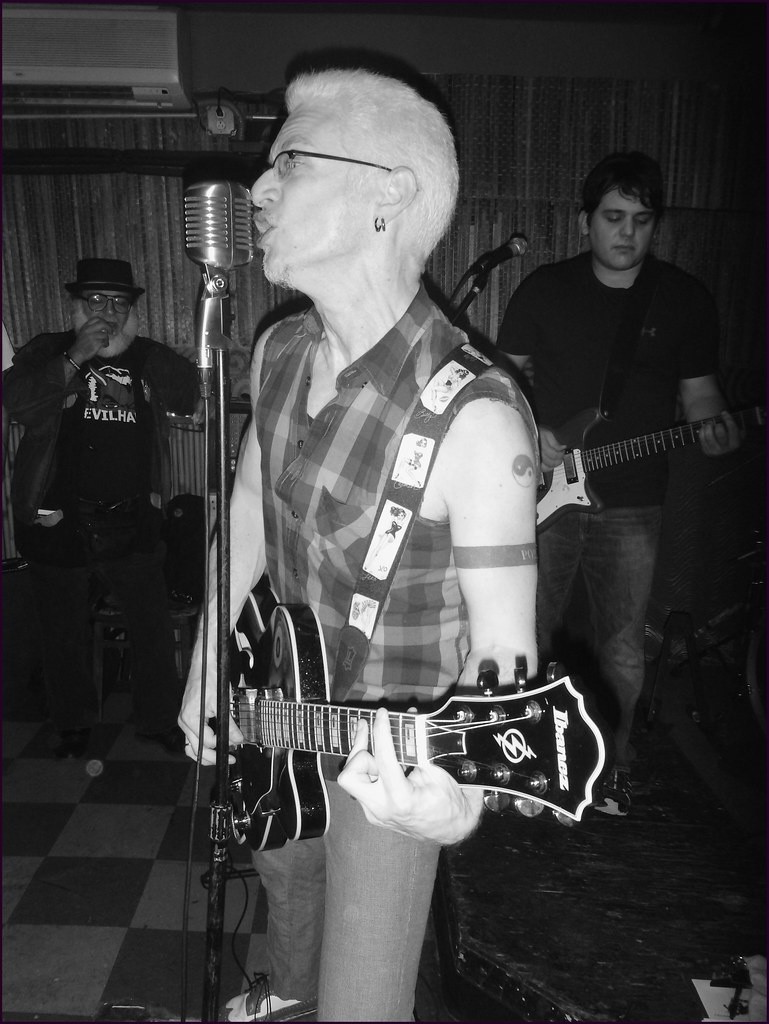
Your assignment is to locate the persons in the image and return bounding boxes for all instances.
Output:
[176,71,540,1022]
[496,150,741,813]
[2,257,198,763]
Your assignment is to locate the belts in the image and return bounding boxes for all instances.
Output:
[82,492,145,518]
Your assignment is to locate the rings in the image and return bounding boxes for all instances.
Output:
[185,743,191,746]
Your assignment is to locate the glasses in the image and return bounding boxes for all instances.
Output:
[71,289,137,315]
[270,149,393,180]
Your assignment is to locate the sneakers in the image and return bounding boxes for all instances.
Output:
[598,759,634,819]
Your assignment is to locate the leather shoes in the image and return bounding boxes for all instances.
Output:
[47,723,90,766]
[138,726,187,758]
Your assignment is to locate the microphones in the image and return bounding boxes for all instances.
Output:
[474,237,528,273]
[185,178,254,268]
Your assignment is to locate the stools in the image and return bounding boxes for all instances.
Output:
[90,605,200,721]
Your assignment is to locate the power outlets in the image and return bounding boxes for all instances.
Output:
[206,106,234,136]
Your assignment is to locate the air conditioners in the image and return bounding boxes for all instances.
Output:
[2,2,194,110]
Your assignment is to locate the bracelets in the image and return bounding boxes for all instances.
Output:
[63,352,81,371]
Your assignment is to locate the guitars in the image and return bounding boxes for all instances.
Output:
[225,590,606,853]
[535,399,767,533]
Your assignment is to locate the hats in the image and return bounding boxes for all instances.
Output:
[65,258,145,297]
